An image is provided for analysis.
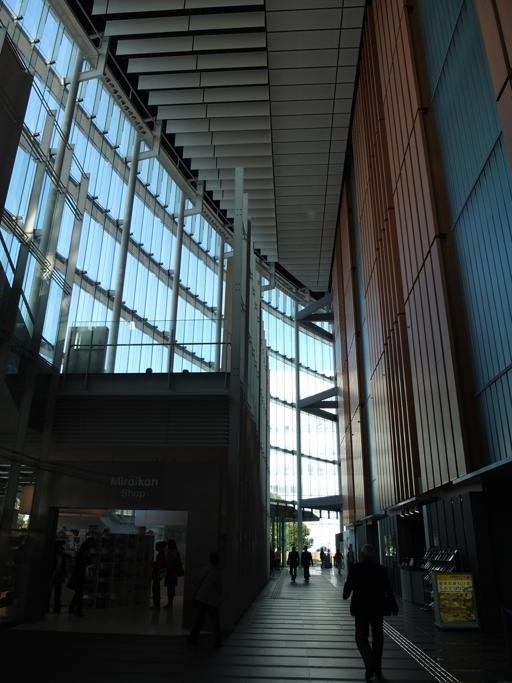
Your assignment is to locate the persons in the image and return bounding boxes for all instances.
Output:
[275,546,282,570]
[286,545,300,581]
[333,547,345,575]
[347,546,356,568]
[187,551,227,648]
[319,546,327,573]
[343,543,400,681]
[301,545,314,581]
[325,548,334,569]
[49,523,185,620]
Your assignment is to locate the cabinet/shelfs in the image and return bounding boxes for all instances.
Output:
[1,525,157,629]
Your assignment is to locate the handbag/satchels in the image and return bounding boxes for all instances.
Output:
[191,590,200,607]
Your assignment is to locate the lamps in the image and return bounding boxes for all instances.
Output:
[399,500,420,518]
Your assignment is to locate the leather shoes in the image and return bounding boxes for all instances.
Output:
[365,666,388,683]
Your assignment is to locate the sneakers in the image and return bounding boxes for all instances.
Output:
[212,641,224,649]
[186,636,198,644]
